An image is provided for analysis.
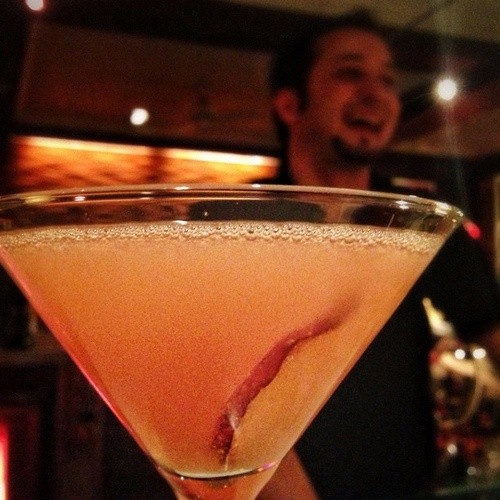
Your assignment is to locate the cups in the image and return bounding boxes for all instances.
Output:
[0,182,463,500]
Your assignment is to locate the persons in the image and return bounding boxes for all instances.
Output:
[191,8,500,500]
[430,331,499,485]
